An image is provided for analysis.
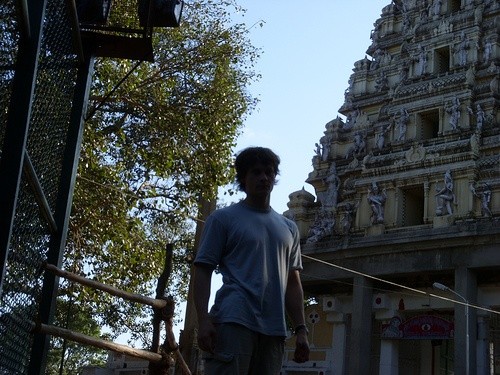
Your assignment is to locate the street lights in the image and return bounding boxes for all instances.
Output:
[434,282,471,375]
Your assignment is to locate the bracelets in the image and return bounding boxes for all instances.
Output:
[291,323,309,337]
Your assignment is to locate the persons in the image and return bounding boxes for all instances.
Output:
[309,210,336,240]
[479,182,495,217]
[434,172,458,215]
[321,163,341,209]
[344,0,500,96]
[475,104,488,130]
[314,143,321,163]
[320,102,392,169]
[367,183,387,224]
[340,201,360,235]
[444,96,463,130]
[393,109,411,142]
[193,146,311,375]
